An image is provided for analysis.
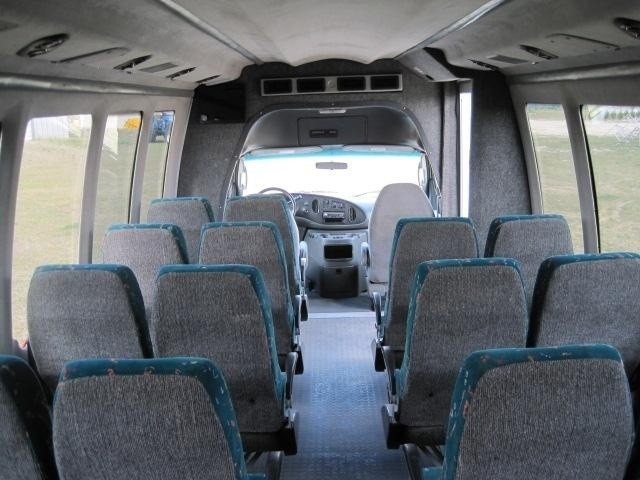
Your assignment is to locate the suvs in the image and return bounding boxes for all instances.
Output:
[149,110,175,142]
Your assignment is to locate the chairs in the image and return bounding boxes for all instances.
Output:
[401,340,635,480]
[101,219,193,357]
[197,221,307,380]
[151,265,302,460]
[221,195,308,328]
[147,195,216,264]
[366,215,481,373]
[376,253,532,453]
[0,352,59,480]
[47,355,270,480]
[26,262,150,413]
[484,210,575,312]
[360,181,436,311]
[529,249,639,368]
